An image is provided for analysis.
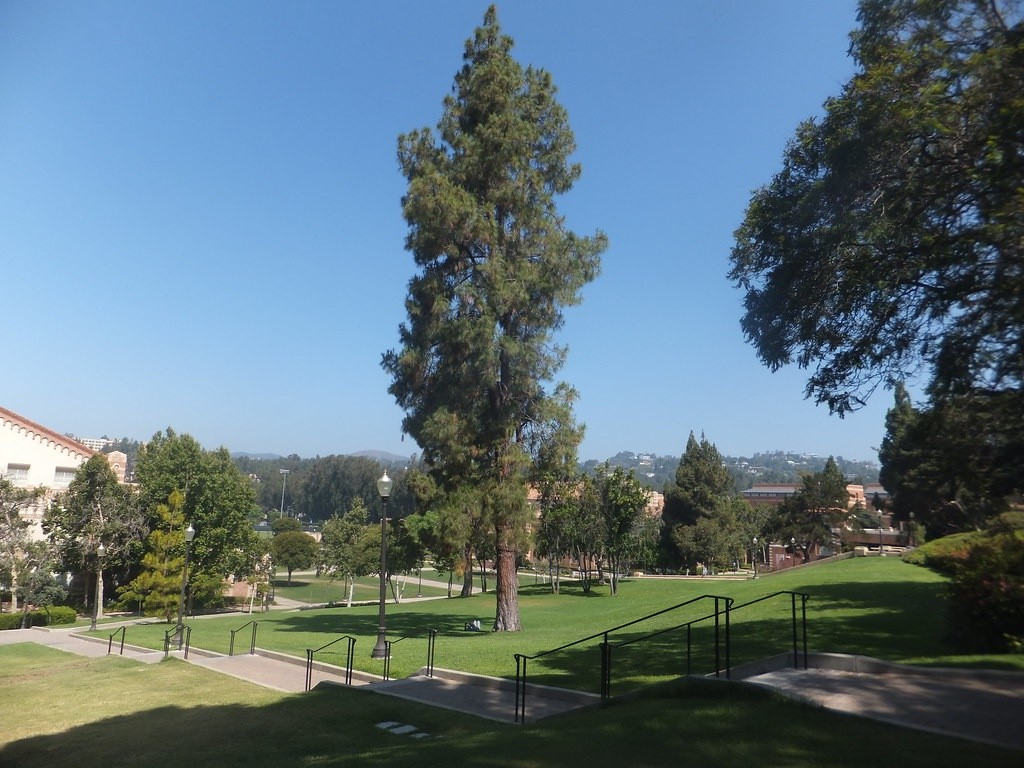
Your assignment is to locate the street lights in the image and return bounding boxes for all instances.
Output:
[790,537,796,566]
[20,565,39,628]
[171,523,197,645]
[752,537,759,577]
[909,511,915,546]
[578,566,581,581]
[419,563,423,595]
[88,543,107,632]
[272,564,277,602]
[277,468,290,519]
[265,565,273,611]
[369,468,393,659]
[877,509,883,550]
